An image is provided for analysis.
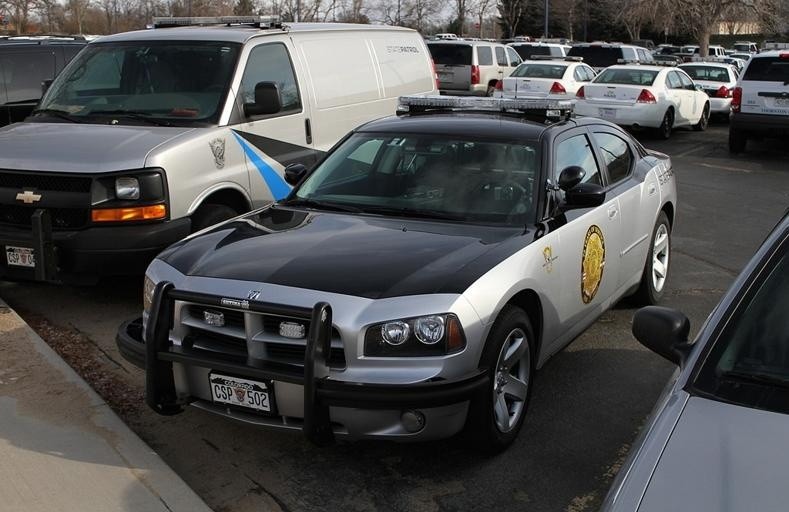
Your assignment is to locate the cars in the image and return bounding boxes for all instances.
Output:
[492,53,598,98]
[503,36,789,123]
[117,93,678,456]
[597,206,789,512]
[572,58,711,140]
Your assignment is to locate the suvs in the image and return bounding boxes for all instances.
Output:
[426,32,526,97]
[728,48,789,146]
[0,34,102,129]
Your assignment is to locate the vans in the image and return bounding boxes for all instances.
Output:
[1,15,440,296]
